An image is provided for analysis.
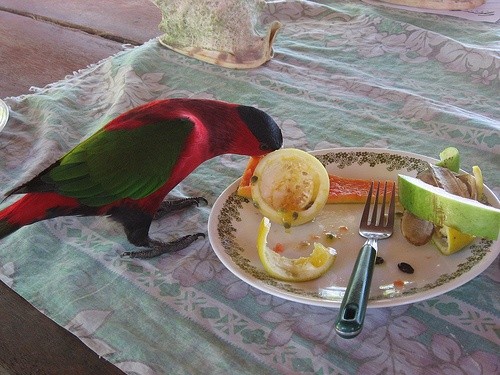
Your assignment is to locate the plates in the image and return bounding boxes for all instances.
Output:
[207,147,500,309]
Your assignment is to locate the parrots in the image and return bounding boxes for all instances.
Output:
[0,98,283,259]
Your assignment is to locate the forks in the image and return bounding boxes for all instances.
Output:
[334,180,396,339]
